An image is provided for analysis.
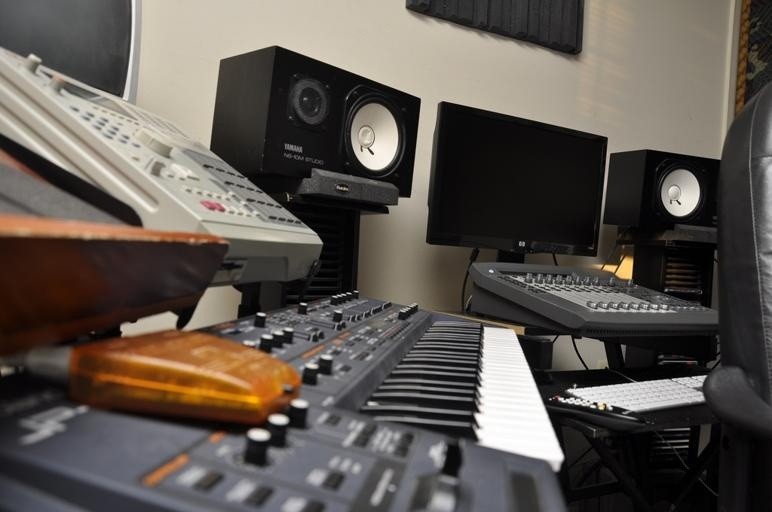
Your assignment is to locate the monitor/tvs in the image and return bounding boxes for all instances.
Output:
[426,100,608,265]
[0,1,142,103]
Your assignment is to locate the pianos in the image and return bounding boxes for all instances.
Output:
[2,290,567,512]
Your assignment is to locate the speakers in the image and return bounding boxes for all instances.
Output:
[210,45,420,200]
[603,148,721,226]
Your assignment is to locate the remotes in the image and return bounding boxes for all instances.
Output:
[545,392,646,421]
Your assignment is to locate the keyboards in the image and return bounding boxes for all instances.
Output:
[565,374,706,413]
